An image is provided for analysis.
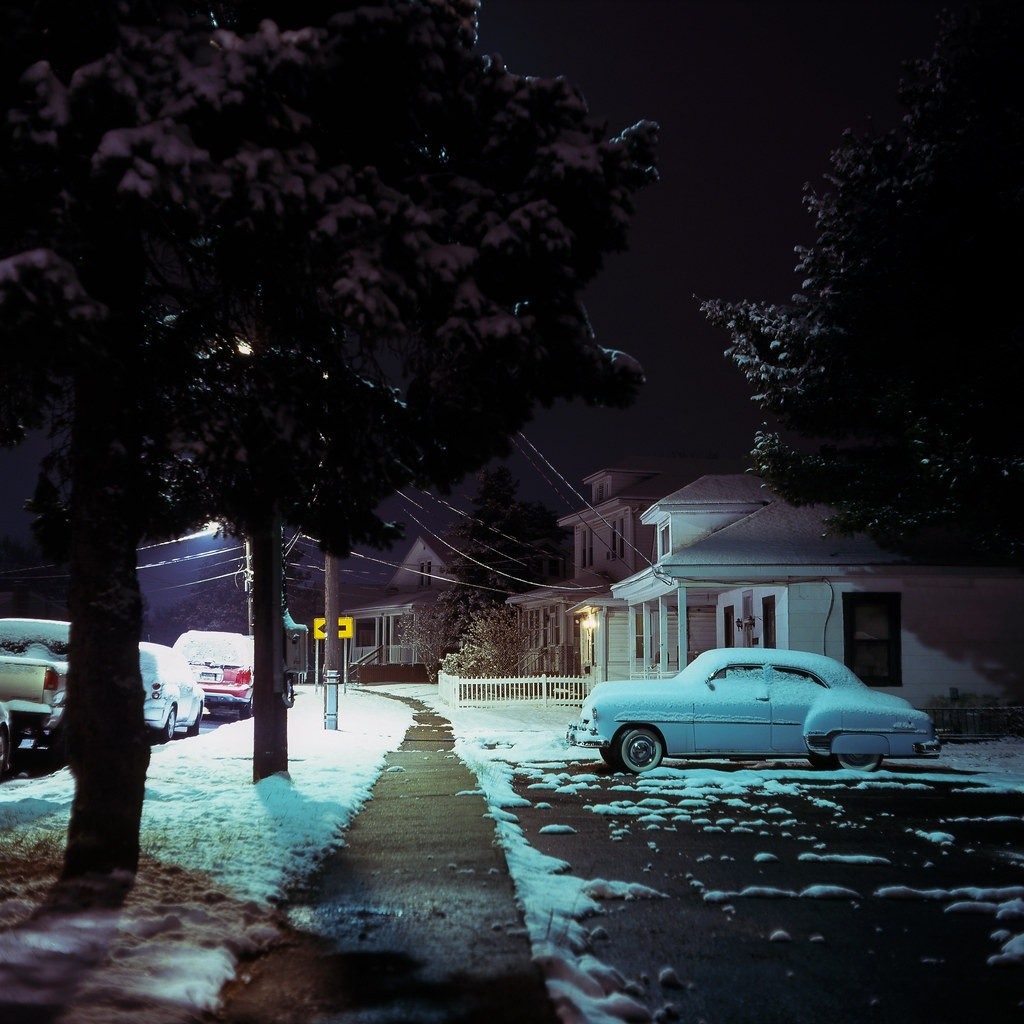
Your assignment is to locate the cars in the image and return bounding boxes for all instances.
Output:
[565,649,941,775]
[139,643,205,743]
[0,615,70,780]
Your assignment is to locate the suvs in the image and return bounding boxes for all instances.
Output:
[171,631,254,721]
